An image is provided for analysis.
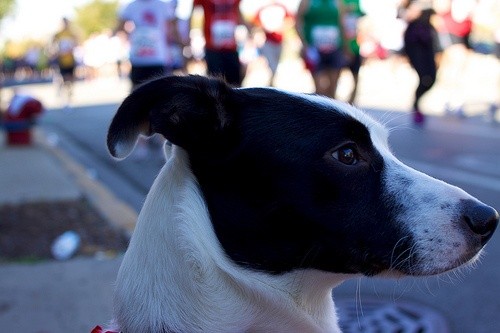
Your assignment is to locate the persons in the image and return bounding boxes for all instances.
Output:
[294,0,349,100]
[1,15,131,108]
[334,0,367,109]
[249,0,291,89]
[162,0,191,77]
[110,0,180,92]
[186,0,252,91]
[357,0,500,125]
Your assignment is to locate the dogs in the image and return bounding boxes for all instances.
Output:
[106,72,500,333]
